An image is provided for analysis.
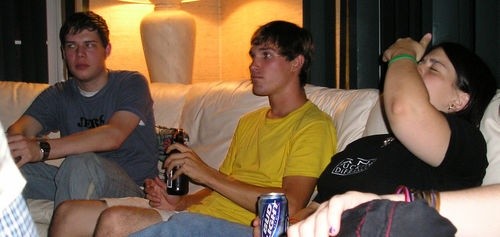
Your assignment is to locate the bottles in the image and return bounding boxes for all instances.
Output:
[167,132,189,196]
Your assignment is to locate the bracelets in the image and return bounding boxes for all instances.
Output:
[389,55,417,64]
[396,186,441,215]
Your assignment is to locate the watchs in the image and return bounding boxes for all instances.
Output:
[38,141,52,161]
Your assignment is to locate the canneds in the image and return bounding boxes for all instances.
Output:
[258,192,290,237]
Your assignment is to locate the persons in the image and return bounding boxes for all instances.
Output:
[288,182,498,237]
[126,34,497,237]
[7,12,161,210]
[0,119,38,237]
[48,20,337,237]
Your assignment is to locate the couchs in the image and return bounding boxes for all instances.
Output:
[0,81,499,236]
